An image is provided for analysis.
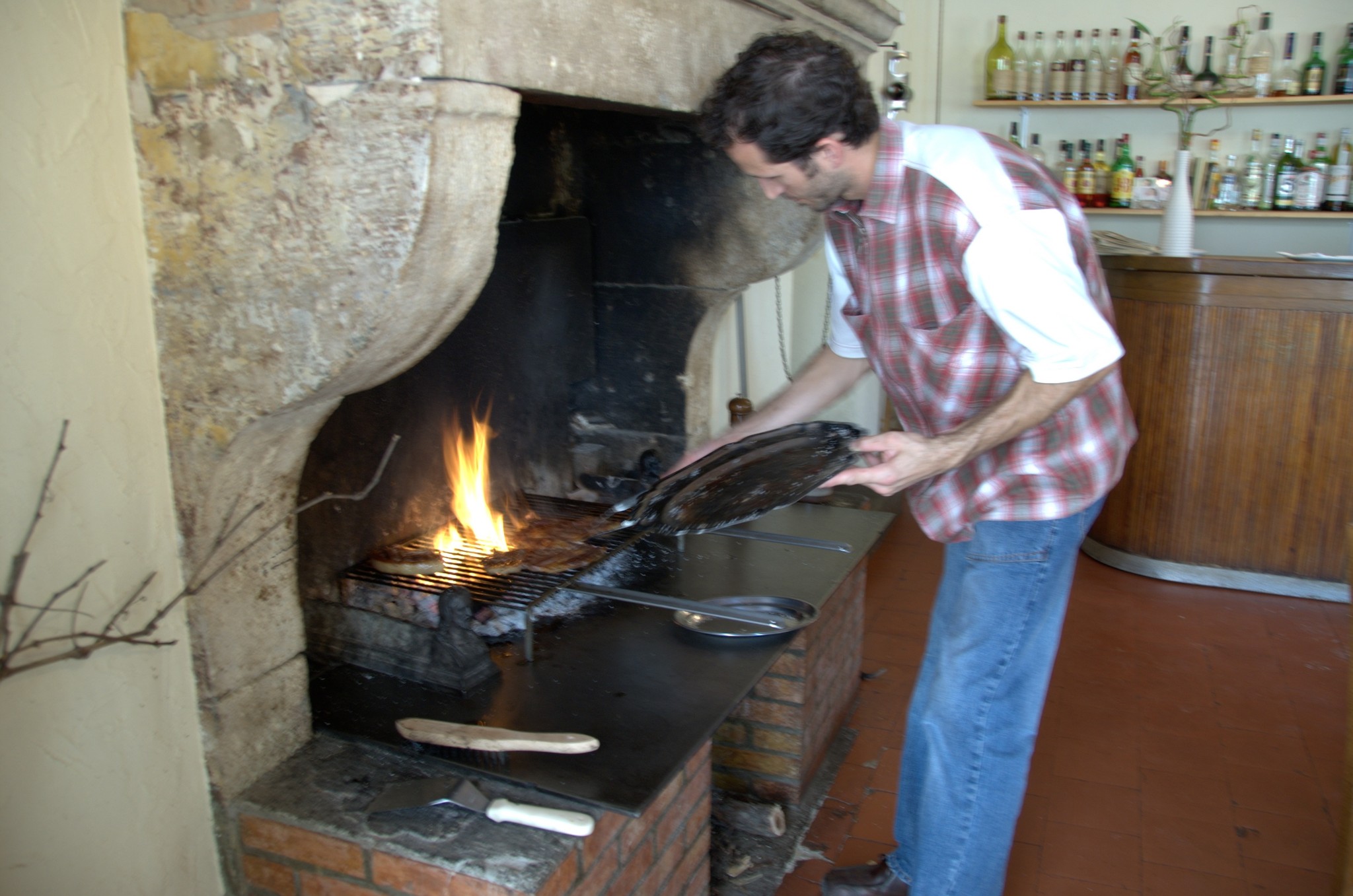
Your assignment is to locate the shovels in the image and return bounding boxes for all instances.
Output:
[368,779,596,836]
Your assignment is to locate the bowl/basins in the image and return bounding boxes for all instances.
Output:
[672,593,822,647]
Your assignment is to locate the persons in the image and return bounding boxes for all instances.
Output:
[660,27,1139,896]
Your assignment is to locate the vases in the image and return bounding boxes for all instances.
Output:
[1162,147,1195,256]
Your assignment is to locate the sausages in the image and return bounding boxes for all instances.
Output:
[368,546,444,575]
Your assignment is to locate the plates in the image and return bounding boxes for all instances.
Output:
[630,421,870,538]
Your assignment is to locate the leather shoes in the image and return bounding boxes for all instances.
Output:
[822,853,910,896]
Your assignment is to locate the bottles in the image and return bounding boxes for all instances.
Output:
[1011,120,1353,211]
[983,10,1353,101]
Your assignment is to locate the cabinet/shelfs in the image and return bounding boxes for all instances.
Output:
[973,92,1353,221]
